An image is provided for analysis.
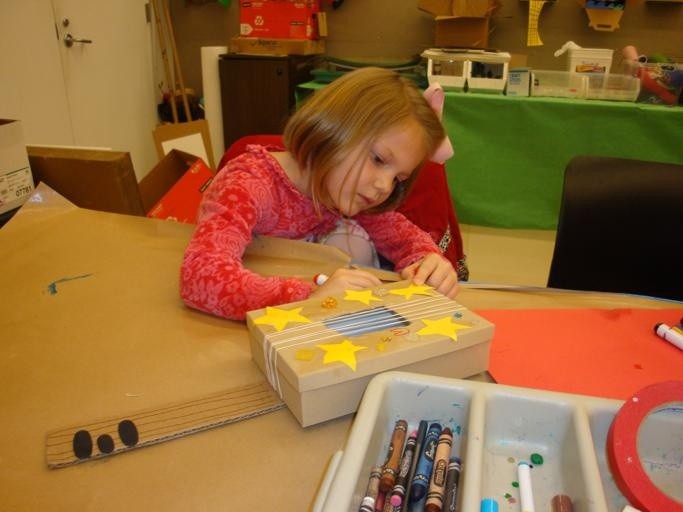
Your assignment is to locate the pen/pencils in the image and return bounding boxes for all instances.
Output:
[553,494,576,512]
[654,317,683,351]
[359,419,461,512]
[479,499,499,512]
[517,461,534,512]
[313,273,330,286]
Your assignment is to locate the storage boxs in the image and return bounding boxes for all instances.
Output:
[0,118,35,217]
[239,0,323,41]
[416,1,496,50]
[136,148,216,225]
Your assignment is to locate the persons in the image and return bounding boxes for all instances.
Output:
[179,66,461,323]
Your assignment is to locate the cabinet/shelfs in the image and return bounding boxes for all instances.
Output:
[217,53,316,152]
[0,0,168,181]
